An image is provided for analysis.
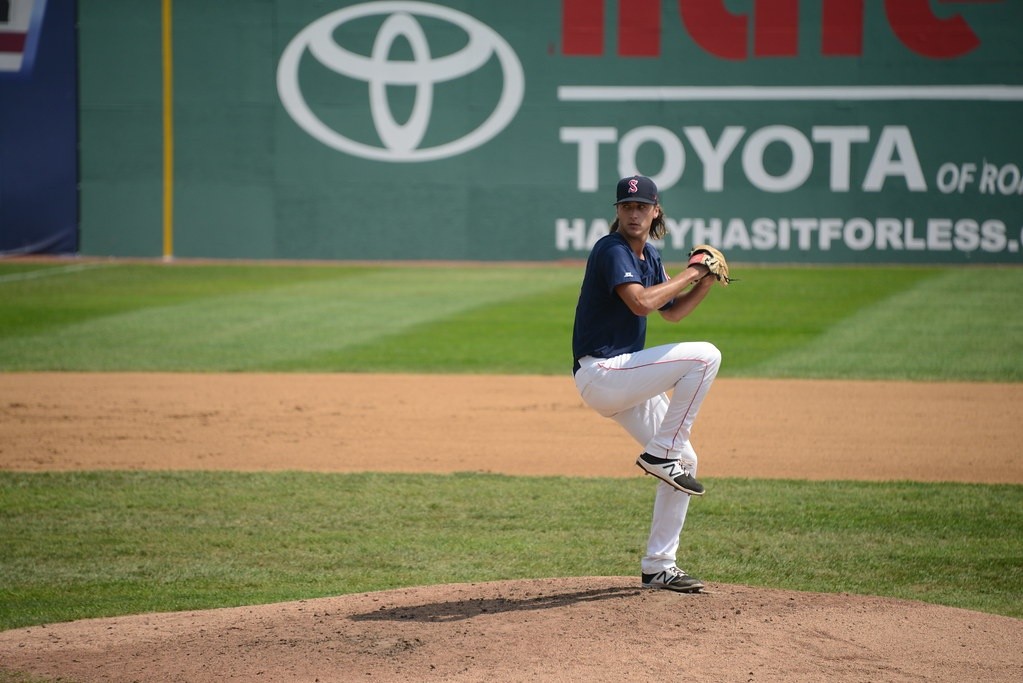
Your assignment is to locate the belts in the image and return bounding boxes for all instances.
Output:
[573,363,582,376]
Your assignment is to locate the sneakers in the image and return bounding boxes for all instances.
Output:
[642,566,704,593]
[636,452,706,496]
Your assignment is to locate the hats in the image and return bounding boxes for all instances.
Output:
[613,175,657,205]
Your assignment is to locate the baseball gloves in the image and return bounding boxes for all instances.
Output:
[686,244,741,288]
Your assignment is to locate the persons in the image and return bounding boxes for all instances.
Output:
[570,175,730,590]
